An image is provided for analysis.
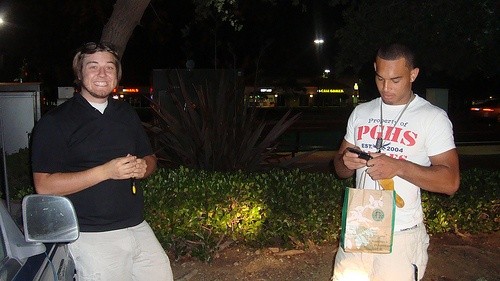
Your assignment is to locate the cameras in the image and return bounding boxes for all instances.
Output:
[346,147,373,161]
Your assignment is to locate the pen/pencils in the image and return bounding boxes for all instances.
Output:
[132,178,136,194]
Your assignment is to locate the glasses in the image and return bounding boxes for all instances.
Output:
[80,41,117,55]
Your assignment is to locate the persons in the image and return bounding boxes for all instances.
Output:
[30,41,174,281]
[330,40,460,281]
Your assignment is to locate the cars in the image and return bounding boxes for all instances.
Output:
[466,100,500,122]
[0,194,79,281]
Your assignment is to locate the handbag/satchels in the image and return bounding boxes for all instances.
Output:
[340,170,395,254]
